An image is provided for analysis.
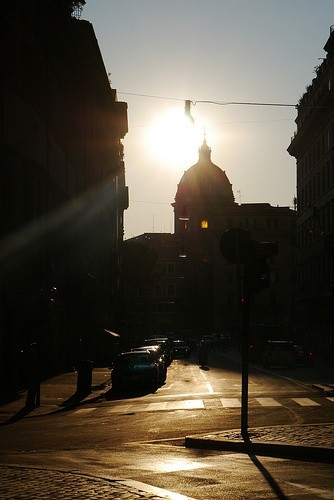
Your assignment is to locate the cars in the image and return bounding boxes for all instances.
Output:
[110,336,191,392]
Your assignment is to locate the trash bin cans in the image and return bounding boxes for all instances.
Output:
[77,359,93,395]
